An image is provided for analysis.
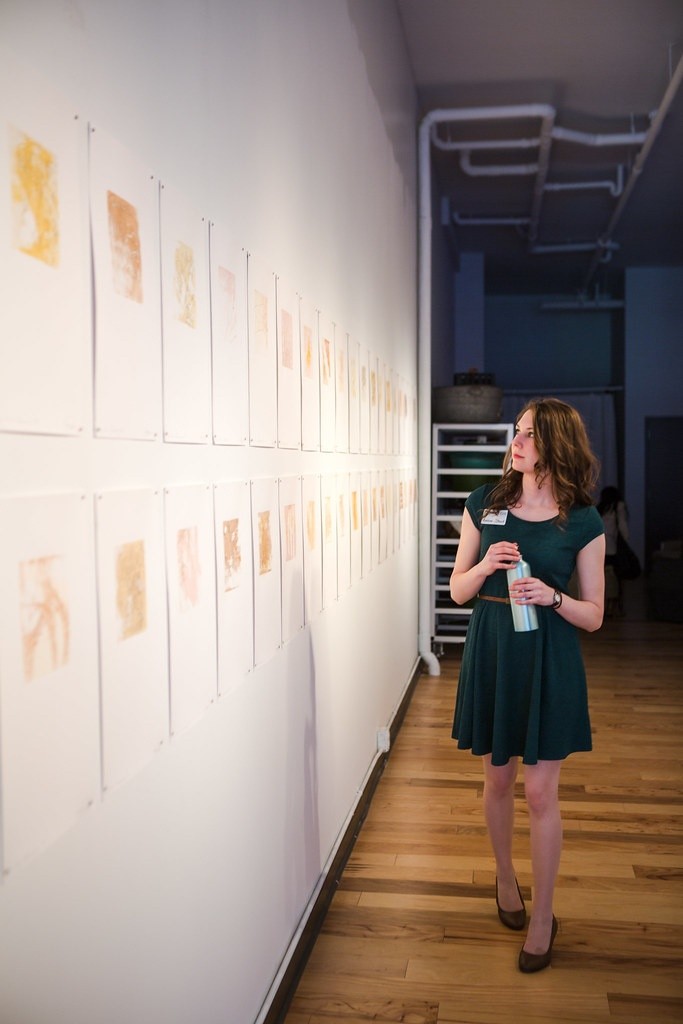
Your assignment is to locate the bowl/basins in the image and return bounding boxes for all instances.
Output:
[432,384,504,424]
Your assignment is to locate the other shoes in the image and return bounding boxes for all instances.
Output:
[517,913,557,973]
[493,876,527,930]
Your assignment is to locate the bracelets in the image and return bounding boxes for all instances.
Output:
[552,592,562,609]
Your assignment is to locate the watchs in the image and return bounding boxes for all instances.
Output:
[549,589,561,608]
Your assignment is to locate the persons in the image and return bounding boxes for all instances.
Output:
[450,398,606,974]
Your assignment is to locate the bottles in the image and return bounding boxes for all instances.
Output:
[506,554,539,632]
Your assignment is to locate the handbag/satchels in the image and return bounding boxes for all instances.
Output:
[613,536,641,580]
